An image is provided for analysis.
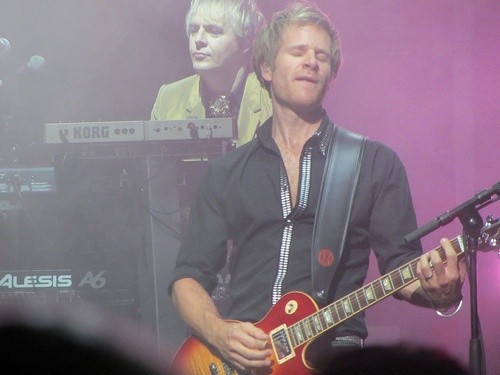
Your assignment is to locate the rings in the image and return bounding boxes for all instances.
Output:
[425,273,432,281]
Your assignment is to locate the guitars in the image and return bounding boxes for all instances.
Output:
[170,213,500,375]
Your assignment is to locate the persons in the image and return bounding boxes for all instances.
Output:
[167,4,466,372]
[150,0,273,147]
[314,343,469,375]
[0,313,164,375]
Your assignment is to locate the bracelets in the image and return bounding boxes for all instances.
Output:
[432,296,463,318]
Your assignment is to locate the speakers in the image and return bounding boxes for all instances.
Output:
[0,166,62,313]
[56,148,235,354]
